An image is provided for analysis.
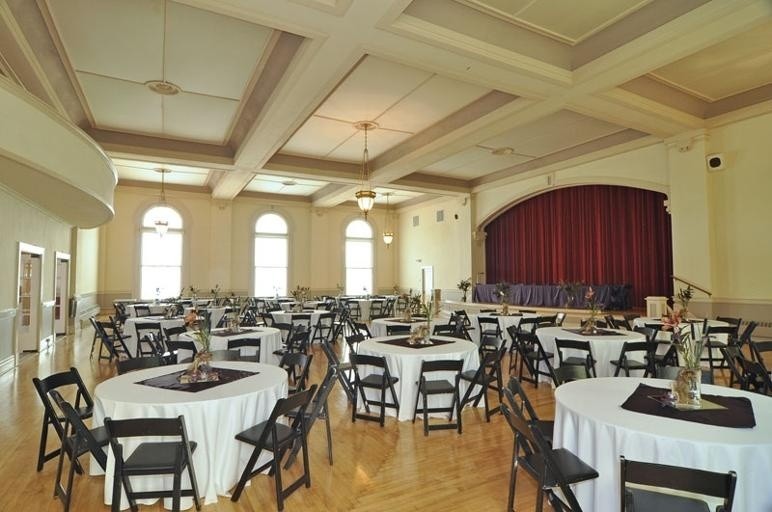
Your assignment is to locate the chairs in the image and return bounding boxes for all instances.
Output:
[228,382,319,511]
[413,358,465,436]
[281,362,340,471]
[49,390,109,512]
[500,375,554,448]
[499,402,599,512]
[102,413,204,512]
[88,293,542,403]
[618,453,738,512]
[347,352,402,427]
[504,296,770,397]
[451,347,508,423]
[32,366,95,477]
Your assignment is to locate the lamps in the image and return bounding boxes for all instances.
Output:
[155,168,171,237]
[355,122,378,221]
[382,192,394,249]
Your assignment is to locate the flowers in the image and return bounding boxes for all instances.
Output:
[583,285,606,316]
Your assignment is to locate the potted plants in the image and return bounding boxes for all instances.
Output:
[665,325,709,412]
[458,280,471,302]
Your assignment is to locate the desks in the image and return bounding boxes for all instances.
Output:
[473,284,632,311]
